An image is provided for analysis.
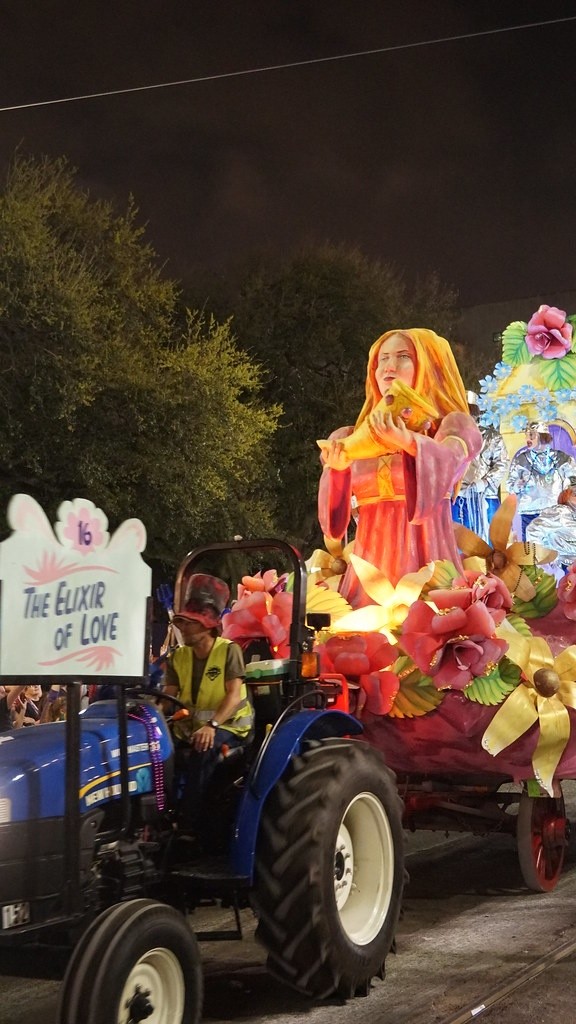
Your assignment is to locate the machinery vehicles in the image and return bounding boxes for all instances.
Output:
[0,536,409,1024]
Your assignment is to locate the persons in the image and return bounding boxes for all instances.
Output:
[525,475,576,586]
[158,575,254,844]
[149,624,175,689]
[506,421,576,542]
[450,390,508,550]
[0,682,100,734]
[318,328,481,607]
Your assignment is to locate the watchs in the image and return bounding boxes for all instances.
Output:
[206,719,219,728]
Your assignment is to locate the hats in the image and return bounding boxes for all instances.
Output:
[171,574,229,637]
[465,390,479,406]
[524,421,550,434]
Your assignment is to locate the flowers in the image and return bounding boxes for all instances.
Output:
[525,304,573,361]
[225,566,576,798]
